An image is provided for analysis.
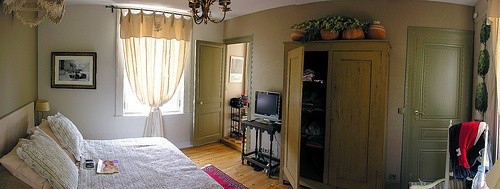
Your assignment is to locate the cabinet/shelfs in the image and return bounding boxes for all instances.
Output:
[229,104,248,140]
[281,40,392,189]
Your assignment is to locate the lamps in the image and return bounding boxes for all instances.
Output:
[34,99,50,118]
[188,0,232,25]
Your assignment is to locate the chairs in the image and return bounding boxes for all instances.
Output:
[409,120,490,189]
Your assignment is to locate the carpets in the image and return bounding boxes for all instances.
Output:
[202,164,250,189]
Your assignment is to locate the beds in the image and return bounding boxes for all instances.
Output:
[0,101,224,189]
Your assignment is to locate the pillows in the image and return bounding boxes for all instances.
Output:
[0,112,84,189]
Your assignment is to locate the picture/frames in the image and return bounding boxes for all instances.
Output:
[50,51,98,90]
[229,55,245,83]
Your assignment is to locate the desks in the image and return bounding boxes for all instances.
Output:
[241,119,281,178]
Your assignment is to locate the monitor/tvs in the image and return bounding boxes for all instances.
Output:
[254,91,281,119]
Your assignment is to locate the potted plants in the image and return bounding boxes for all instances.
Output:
[290,14,370,41]
